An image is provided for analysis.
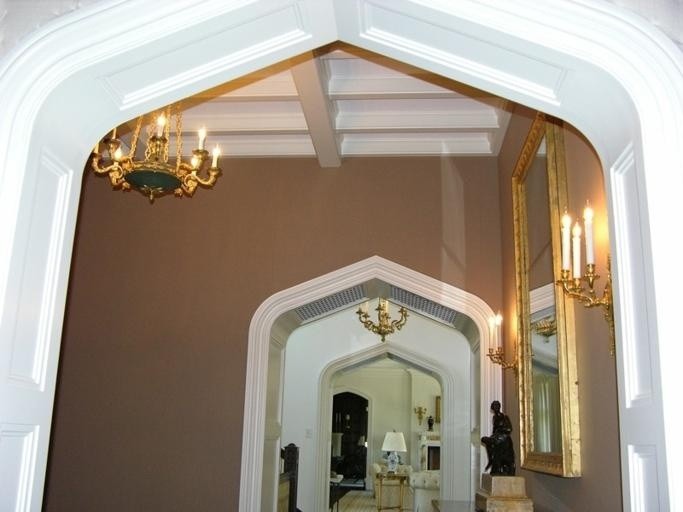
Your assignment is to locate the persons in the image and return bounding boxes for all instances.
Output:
[482,401,512,471]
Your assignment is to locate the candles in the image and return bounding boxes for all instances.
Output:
[489,315,494,349]
[562,205,595,279]
[496,311,502,347]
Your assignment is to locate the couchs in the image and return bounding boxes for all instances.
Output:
[411,471,440,512]
[373,464,412,508]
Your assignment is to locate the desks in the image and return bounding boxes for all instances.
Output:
[377,473,406,512]
[330,475,344,512]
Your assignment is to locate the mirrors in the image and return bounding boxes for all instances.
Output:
[512,111,581,479]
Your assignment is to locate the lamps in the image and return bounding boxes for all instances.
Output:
[382,432,407,472]
[92,102,222,202]
[355,298,409,342]
